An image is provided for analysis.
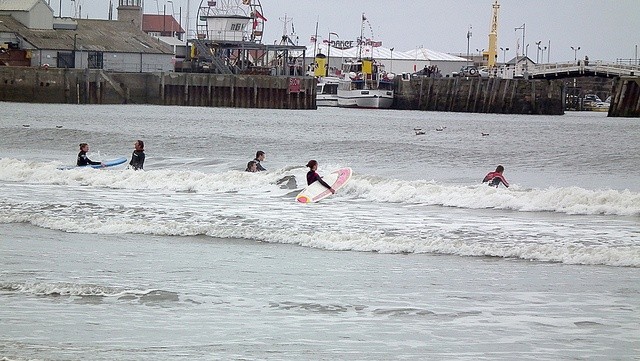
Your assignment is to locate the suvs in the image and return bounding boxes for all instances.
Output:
[463,65,477,76]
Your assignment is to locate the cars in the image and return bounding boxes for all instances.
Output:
[479,69,488,78]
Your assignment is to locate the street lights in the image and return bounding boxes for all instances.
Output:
[571,46,580,60]
[536,40,541,61]
[526,43,529,56]
[467,30,472,64]
[476,48,484,68]
[500,47,509,65]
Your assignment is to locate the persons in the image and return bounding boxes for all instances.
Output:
[305,160,335,195]
[493,63,497,69]
[125,140,145,172]
[423,65,427,73]
[489,63,492,71]
[432,65,434,72]
[428,66,431,72]
[77,143,106,168]
[246,159,258,171]
[482,165,509,189]
[585,56,589,65]
[525,69,528,79]
[435,64,439,72]
[521,69,524,77]
[254,151,267,171]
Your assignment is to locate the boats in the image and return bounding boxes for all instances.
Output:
[305,63,340,108]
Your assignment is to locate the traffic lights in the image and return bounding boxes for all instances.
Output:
[336,57,394,108]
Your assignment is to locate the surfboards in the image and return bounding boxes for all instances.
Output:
[58,156,127,171]
[296,167,352,202]
